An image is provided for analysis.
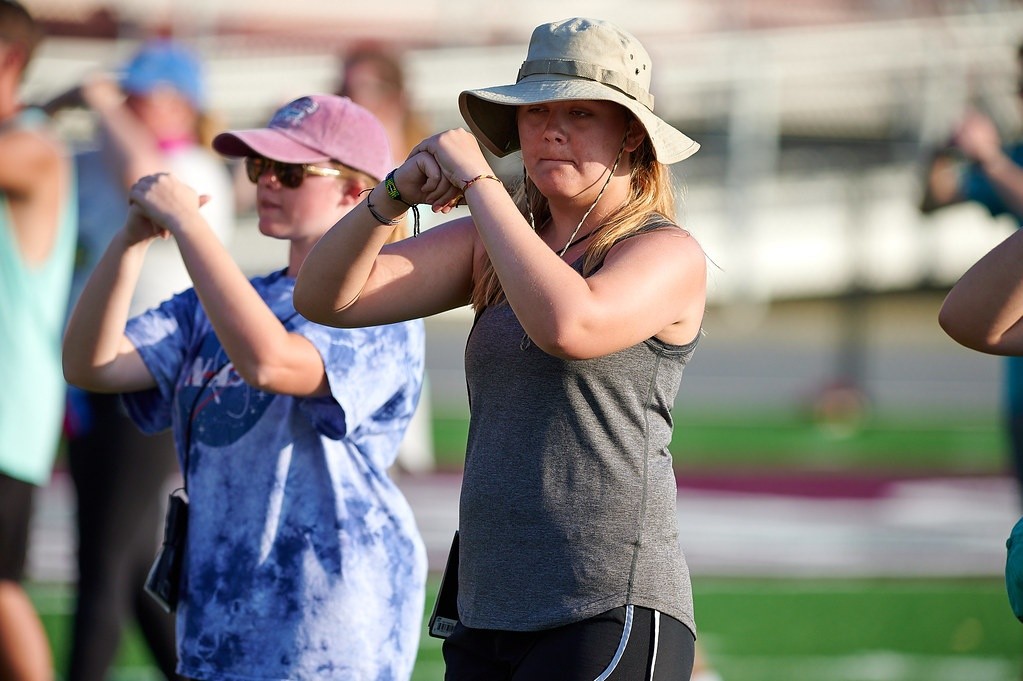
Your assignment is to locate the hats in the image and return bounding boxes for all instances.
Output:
[214,94,392,182]
[459,18,700,164]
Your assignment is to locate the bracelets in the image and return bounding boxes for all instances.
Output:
[357,188,408,225]
[386,170,420,238]
[450,174,503,208]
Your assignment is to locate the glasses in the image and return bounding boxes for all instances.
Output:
[245,152,347,189]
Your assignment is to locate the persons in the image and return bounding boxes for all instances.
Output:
[336,46,434,475]
[295,17,707,681]
[923,100,1022,226]
[939,227,1023,624]
[0,0,78,681]
[47,42,238,681]
[61,94,429,681]
